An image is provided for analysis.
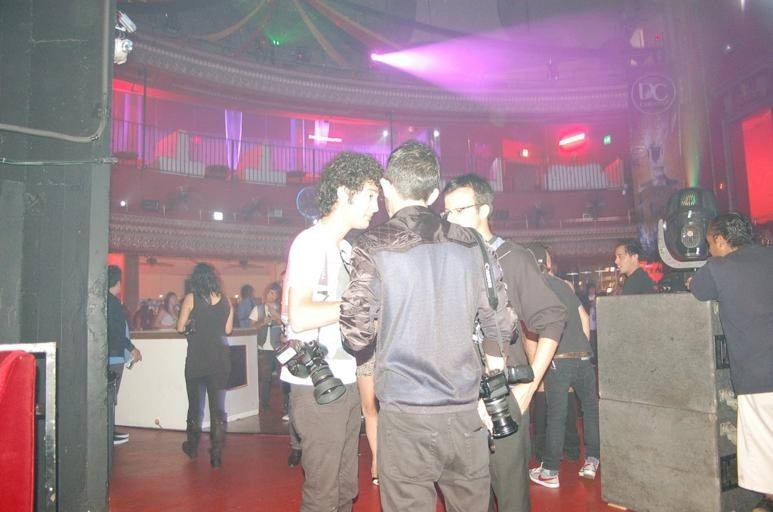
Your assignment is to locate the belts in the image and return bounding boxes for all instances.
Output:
[553,352,590,359]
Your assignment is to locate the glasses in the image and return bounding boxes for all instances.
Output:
[440,205,474,218]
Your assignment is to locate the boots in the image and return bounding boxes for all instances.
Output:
[211,413,227,467]
[183,409,204,458]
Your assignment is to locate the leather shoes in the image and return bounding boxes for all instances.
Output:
[288,450,301,465]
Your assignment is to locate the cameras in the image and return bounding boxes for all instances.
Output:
[287,340,346,404]
[480,370,518,439]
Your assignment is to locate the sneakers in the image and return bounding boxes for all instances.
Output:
[114,432,129,444]
[529,462,560,488]
[578,456,599,480]
